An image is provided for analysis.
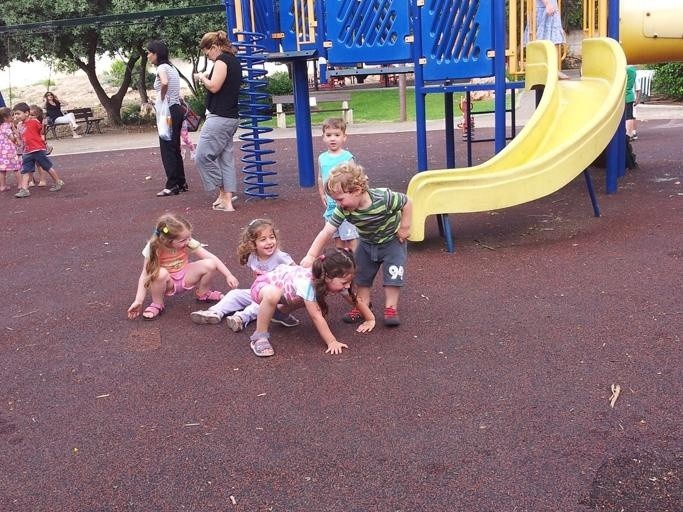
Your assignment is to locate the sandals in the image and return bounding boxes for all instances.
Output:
[271,309,301,328]
[249,331,275,357]
[212,197,236,212]
[142,302,166,320]
[557,69,573,82]
[195,290,226,303]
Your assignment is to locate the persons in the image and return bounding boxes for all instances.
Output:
[0,91,82,197]
[625,66,641,141]
[193,30,242,212]
[250,247,376,357]
[179,98,197,160]
[523,0,572,80]
[127,212,240,321]
[191,219,297,332]
[317,117,360,252]
[147,42,188,196]
[300,163,412,326]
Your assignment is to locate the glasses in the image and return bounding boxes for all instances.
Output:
[204,47,212,57]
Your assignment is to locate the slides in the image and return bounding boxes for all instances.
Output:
[403,37,628,243]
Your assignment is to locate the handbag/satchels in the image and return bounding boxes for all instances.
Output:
[179,97,201,132]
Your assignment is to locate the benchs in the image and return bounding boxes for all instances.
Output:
[43,107,104,141]
[272,91,354,129]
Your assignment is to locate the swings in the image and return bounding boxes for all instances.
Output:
[99,23,201,131]
[8,34,56,156]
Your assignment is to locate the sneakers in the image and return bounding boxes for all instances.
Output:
[626,131,641,142]
[38,177,47,188]
[0,184,11,193]
[180,147,187,161]
[15,190,31,198]
[189,149,198,161]
[384,305,401,327]
[189,310,222,326]
[72,133,84,140]
[225,316,243,333]
[341,302,373,324]
[49,180,65,192]
[16,177,35,190]
[72,124,83,132]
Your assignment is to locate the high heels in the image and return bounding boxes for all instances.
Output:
[156,181,190,197]
